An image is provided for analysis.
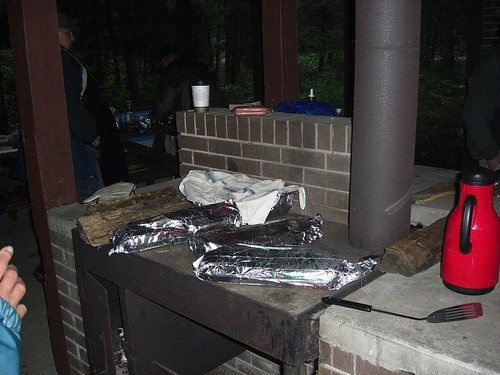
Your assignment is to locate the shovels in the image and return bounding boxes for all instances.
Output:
[321,295,483,323]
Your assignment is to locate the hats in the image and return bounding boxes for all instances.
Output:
[58,12,80,34]
[159,46,177,58]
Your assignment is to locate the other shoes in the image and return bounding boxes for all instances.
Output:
[34,262,44,282]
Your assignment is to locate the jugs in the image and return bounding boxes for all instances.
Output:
[439,167,500,295]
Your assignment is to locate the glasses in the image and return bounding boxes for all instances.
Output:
[59,29,72,35]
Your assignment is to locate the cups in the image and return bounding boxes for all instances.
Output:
[191,85,210,108]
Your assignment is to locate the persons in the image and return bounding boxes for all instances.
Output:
[463,46,500,171]
[14,12,228,282]
[0,245,28,375]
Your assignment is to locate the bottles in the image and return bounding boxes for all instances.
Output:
[125,101,136,124]
[308,89,316,102]
